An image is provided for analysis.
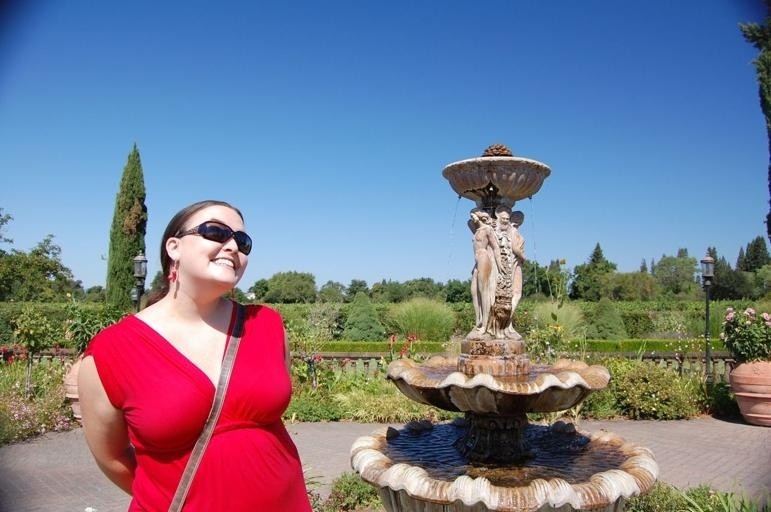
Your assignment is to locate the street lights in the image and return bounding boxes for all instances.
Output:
[701,258,715,409]
[133,250,148,313]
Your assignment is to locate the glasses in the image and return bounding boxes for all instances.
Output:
[172,220,252,256]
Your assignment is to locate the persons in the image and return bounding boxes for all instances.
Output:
[505,211,528,340]
[76,201,313,511]
[469,208,507,336]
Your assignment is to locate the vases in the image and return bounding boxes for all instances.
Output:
[729,357,771,427]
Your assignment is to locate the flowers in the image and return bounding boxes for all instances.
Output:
[719,307,771,363]
